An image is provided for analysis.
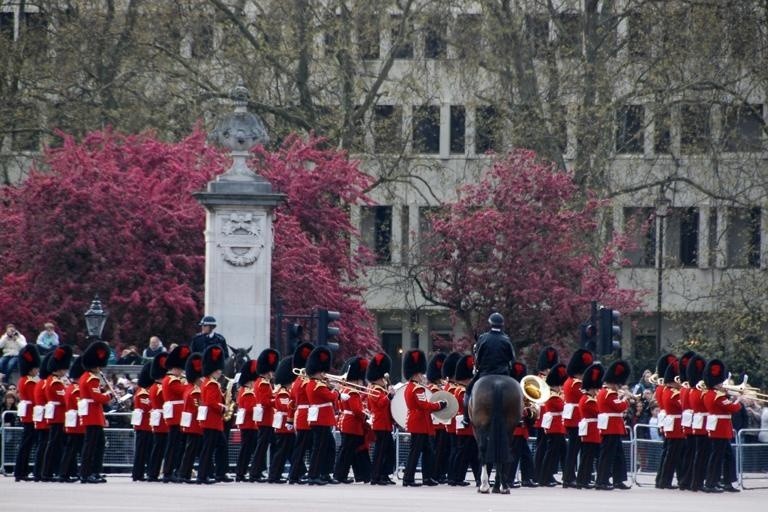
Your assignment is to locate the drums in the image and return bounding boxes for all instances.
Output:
[389,383,434,429]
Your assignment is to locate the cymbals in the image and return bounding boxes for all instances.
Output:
[430,391,459,418]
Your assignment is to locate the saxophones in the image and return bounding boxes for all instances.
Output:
[222,373,235,422]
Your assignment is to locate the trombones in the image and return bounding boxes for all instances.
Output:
[649,372,768,402]
[292,365,380,398]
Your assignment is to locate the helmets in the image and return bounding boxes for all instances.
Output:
[198,315,217,327]
[487,312,505,331]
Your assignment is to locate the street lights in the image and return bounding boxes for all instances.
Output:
[652,181,671,359]
[83,294,110,341]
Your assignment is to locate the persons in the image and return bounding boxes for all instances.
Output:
[458,312,526,425]
[142,335,166,359]
[36,321,59,355]
[0,344,767,492]
[190,315,228,358]
[0,322,28,383]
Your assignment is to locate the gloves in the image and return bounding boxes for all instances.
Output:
[284,423,294,431]
[439,400,447,409]
[340,393,351,401]
[385,387,395,400]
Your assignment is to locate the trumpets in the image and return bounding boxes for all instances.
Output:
[617,390,642,403]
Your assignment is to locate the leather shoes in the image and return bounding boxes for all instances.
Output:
[132,473,397,486]
[13,475,108,484]
[654,482,741,493]
[508,477,632,490]
[402,476,471,487]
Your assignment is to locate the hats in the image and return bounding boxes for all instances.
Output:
[402,349,427,380]
[510,362,528,380]
[545,363,569,387]
[567,349,631,390]
[136,344,226,389]
[18,341,111,376]
[426,351,476,384]
[340,355,368,382]
[238,342,332,386]
[654,350,726,388]
[536,346,559,372]
[367,352,393,382]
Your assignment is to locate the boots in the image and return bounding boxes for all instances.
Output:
[461,408,470,428]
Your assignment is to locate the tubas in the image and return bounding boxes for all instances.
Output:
[520,374,551,403]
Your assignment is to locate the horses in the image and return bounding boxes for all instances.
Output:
[222,344,253,391]
[467,374,522,495]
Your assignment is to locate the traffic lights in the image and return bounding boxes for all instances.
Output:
[292,325,303,349]
[322,309,341,352]
[603,309,620,353]
[582,325,598,351]
